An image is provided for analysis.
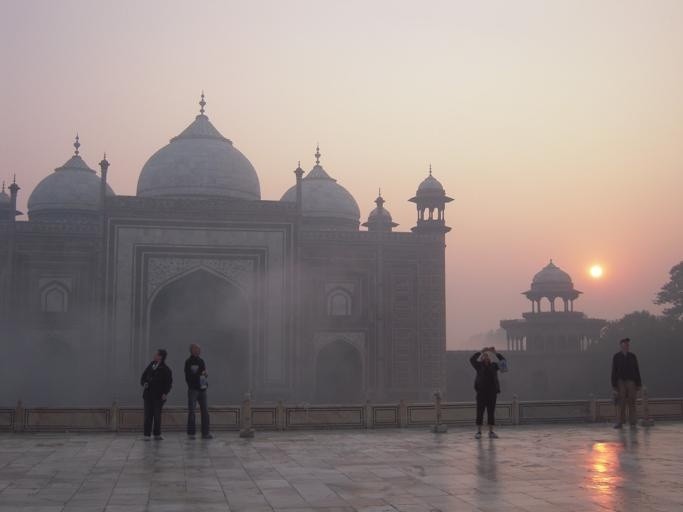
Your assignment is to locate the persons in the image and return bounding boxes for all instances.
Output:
[139,348,174,443]
[183,343,214,440]
[469,346,510,440]
[610,337,642,430]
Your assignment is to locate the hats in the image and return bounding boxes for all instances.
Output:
[620,338,629,344]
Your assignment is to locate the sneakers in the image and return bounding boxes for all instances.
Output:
[144,436,150,441]
[487,432,497,438]
[475,432,480,439]
[153,435,163,440]
[613,423,622,429]
[187,434,194,440]
[201,434,212,439]
